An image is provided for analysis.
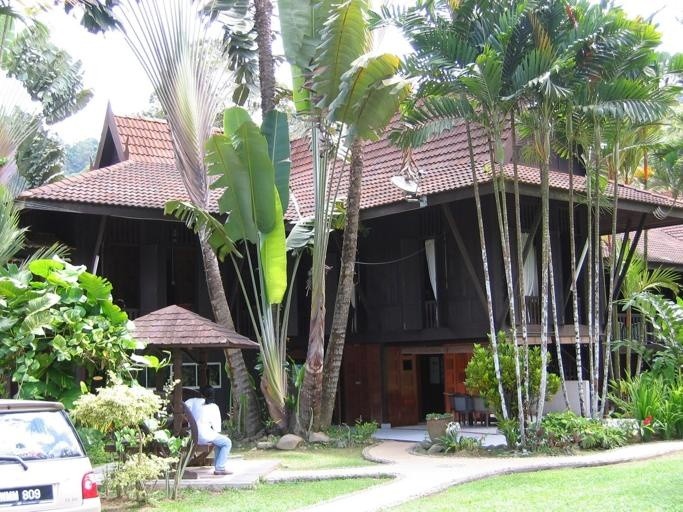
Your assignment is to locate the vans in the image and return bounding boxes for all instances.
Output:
[0,398,102,511]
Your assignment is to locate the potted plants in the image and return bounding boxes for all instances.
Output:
[425,412,454,446]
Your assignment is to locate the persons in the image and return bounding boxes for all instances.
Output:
[183,384,232,475]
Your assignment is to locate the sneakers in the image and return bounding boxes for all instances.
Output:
[214,471,232,474]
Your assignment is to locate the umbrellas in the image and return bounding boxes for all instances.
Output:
[123,303,261,472]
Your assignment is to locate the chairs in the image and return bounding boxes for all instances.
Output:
[447,391,500,427]
[104,387,214,467]
